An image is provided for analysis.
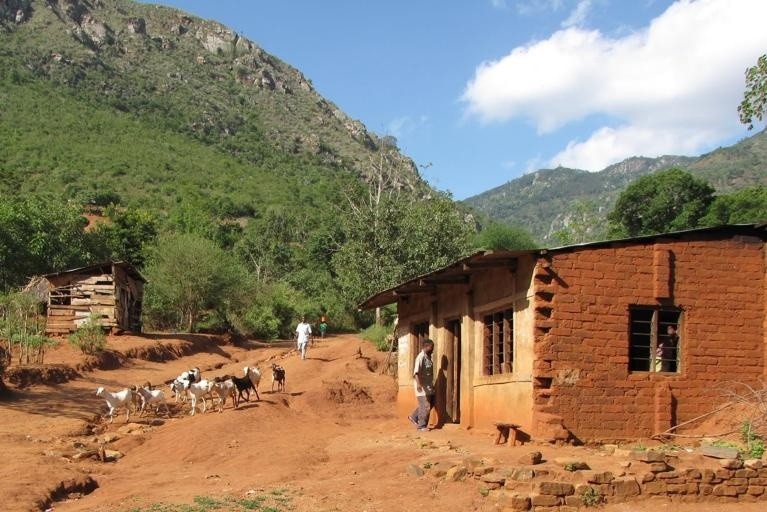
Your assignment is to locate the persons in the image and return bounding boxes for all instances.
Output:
[408,339,434,432]
[293,316,314,360]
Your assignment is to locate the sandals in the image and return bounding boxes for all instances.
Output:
[407,416,430,432]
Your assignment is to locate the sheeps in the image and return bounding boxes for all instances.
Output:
[94,381,169,418]
[170,364,263,416]
[269,362,286,392]
